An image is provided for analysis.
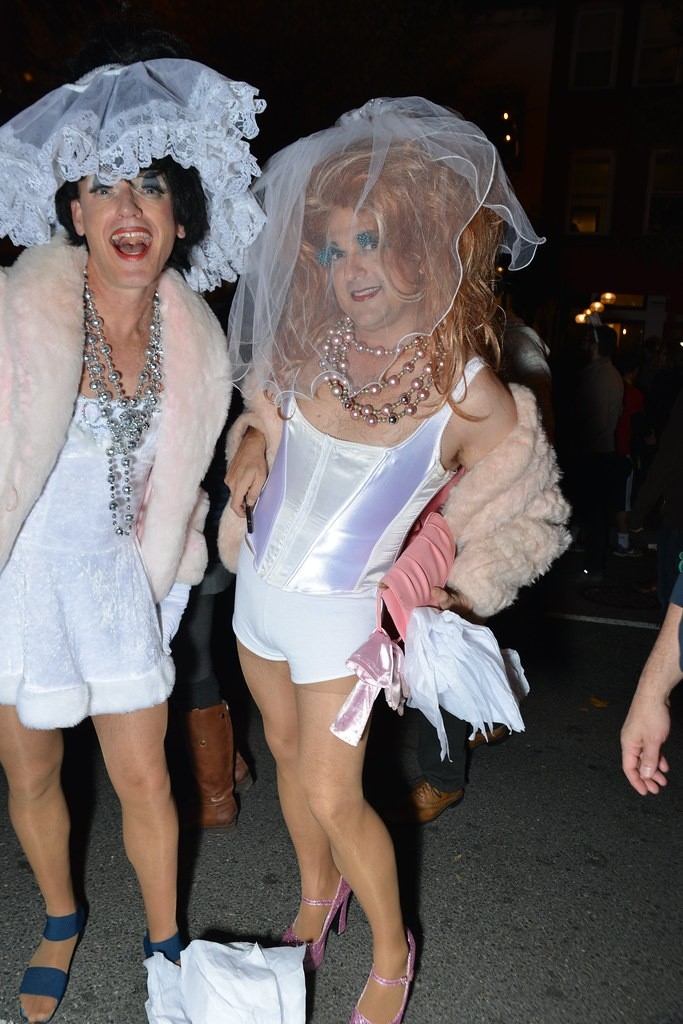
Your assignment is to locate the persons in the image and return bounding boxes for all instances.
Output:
[175,449,251,829]
[0,58,268,1023]
[389,284,683,823]
[218,96,572,1024]
[621,571,683,796]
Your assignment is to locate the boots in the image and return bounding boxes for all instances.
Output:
[174,698,239,833]
[167,700,253,792]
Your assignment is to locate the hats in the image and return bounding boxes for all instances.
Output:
[582,324,617,349]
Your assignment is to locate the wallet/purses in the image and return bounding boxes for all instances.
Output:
[379,509,458,643]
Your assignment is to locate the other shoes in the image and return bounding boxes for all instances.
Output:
[566,539,585,552]
[612,544,644,557]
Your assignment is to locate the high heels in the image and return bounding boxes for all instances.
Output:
[279,872,353,971]
[350,928,416,1024]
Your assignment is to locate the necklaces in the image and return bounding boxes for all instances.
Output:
[84,270,163,535]
[320,316,441,425]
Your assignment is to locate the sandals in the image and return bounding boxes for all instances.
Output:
[144,923,185,965]
[17,908,85,1024]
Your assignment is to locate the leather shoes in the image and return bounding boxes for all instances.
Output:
[394,776,465,823]
[464,725,511,752]
